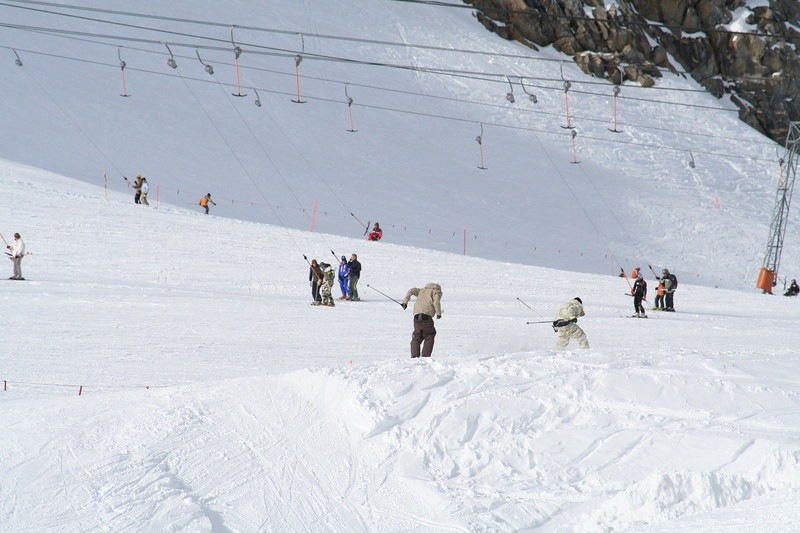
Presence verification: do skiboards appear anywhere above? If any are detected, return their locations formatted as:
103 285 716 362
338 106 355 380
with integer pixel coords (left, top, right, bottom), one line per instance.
626 315 649 318
0 278 26 280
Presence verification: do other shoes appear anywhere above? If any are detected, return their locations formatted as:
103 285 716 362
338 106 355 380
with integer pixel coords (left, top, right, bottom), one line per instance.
352 296 358 300
329 302 334 306
340 295 346 299
311 301 321 305
10 275 21 280
322 302 327 305
347 295 352 300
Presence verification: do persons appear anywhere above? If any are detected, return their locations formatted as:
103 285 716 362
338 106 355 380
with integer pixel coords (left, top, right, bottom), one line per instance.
661 269 678 312
367 222 382 242
132 175 150 205
553 296 590 349
760 282 777 295
199 193 216 215
631 267 641 278
654 281 666 310
632 273 647 318
402 282 443 359
784 278 800 297
6 233 26 280
309 254 361 307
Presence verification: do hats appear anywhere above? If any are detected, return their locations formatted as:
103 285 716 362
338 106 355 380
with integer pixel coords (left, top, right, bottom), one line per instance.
312 260 317 264
341 256 346 261
575 297 581 304
320 262 324 266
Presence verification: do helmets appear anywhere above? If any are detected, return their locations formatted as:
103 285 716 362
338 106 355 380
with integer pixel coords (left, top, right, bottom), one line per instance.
662 269 668 274
638 273 641 276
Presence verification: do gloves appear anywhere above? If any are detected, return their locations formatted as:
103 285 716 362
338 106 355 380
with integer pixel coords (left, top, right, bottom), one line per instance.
437 316 441 319
401 304 407 310
